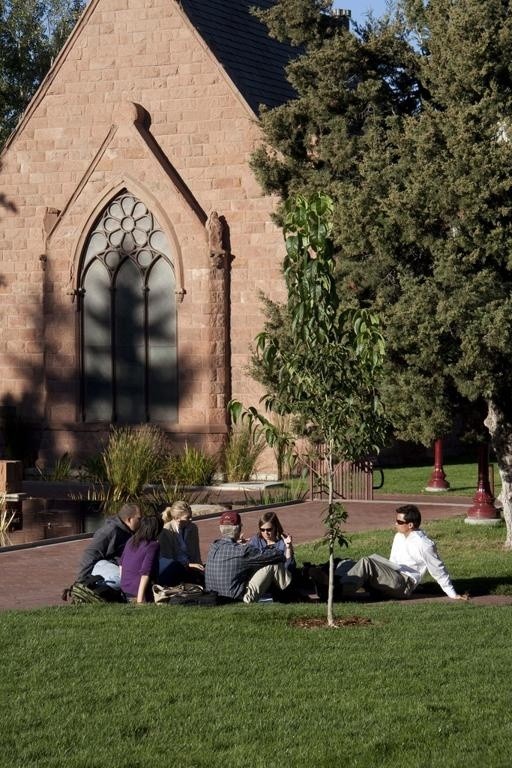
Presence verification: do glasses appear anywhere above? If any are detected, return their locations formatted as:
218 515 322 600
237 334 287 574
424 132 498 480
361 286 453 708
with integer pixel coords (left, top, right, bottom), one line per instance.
396 520 407 525
259 528 273 532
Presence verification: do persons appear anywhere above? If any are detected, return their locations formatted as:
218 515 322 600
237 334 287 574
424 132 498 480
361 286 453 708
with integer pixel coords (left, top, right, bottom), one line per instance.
154 500 204 587
237 511 295 600
203 510 312 604
74 502 142 592
308 503 469 601
119 514 164 605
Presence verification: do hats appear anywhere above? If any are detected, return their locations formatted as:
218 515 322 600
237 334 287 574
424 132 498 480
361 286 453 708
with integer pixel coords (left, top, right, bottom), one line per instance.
219 512 241 526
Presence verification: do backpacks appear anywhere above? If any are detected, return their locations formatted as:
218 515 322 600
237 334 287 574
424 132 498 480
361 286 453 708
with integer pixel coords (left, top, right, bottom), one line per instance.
63 575 128 603
152 584 217 604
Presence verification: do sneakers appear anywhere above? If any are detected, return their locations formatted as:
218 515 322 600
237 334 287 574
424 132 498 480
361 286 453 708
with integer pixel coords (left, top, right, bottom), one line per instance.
308 567 329 584
